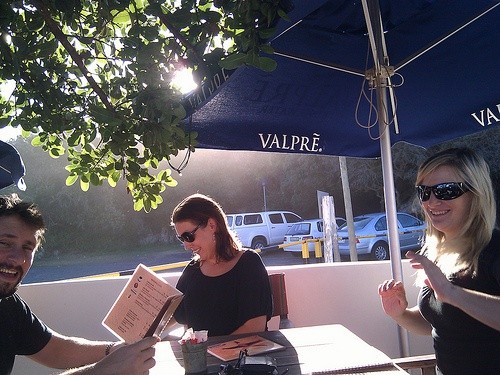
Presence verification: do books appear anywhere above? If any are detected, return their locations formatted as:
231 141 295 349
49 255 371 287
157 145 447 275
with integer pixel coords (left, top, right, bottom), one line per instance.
101 263 186 351
207 335 286 362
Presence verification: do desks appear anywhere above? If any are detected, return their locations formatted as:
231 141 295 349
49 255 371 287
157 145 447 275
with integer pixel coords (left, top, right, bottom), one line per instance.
149 323 410 375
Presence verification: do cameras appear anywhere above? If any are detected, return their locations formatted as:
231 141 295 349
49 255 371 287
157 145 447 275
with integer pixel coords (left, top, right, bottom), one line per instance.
240 357 278 375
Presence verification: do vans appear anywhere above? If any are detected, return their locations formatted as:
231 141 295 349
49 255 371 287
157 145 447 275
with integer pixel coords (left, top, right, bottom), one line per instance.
226 211 303 256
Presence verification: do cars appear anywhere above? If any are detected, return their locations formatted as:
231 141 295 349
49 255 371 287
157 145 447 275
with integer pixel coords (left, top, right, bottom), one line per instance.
337 212 428 260
283 218 346 255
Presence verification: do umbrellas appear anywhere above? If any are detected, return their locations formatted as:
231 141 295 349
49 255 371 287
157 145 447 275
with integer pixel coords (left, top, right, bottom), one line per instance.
144 0 500 356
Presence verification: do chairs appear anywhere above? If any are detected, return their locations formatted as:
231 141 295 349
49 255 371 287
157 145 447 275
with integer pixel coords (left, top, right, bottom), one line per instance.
268 274 289 330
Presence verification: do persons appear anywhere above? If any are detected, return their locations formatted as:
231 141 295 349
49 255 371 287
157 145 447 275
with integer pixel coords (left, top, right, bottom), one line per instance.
0 192 161 375
157 193 274 342
378 147 500 375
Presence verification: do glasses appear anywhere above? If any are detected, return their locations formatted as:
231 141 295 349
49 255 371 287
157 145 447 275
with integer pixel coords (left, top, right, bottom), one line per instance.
415 181 473 202
176 222 206 243
